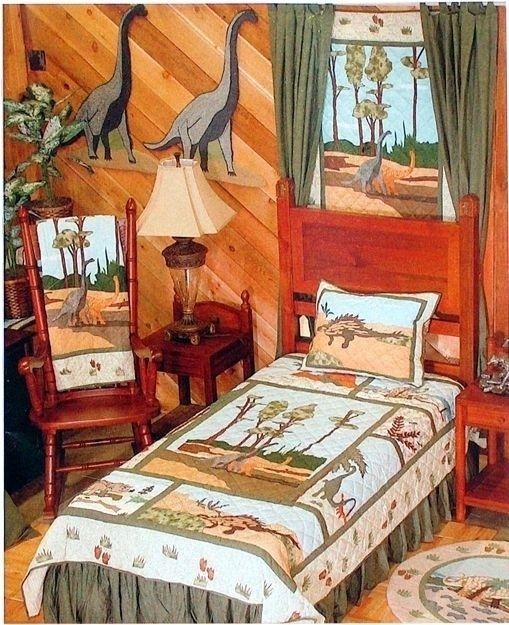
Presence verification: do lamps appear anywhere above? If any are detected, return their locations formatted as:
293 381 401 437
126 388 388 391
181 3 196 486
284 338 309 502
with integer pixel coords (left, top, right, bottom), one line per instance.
136 151 237 346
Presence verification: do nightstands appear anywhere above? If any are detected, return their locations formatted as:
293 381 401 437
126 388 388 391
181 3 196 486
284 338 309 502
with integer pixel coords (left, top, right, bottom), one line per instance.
141 286 255 444
454 379 509 524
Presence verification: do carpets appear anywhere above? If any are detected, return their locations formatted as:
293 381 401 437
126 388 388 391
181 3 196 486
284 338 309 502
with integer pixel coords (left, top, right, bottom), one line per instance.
386 537 509 623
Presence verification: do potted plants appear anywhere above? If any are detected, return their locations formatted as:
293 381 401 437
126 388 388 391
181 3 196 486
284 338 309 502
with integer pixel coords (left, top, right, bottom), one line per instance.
4 160 47 320
3 82 89 225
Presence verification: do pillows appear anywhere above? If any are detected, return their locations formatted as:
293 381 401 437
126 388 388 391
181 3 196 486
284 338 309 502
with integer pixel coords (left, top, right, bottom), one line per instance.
300 278 444 390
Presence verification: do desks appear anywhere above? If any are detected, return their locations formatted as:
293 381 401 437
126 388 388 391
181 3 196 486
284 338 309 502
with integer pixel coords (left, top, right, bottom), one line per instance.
4 316 45 506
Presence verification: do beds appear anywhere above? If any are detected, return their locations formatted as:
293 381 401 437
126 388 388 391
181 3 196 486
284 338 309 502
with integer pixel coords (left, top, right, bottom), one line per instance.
22 177 480 623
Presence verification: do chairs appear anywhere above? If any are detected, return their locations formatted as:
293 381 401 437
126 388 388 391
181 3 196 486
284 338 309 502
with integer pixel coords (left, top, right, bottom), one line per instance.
17 199 162 522
